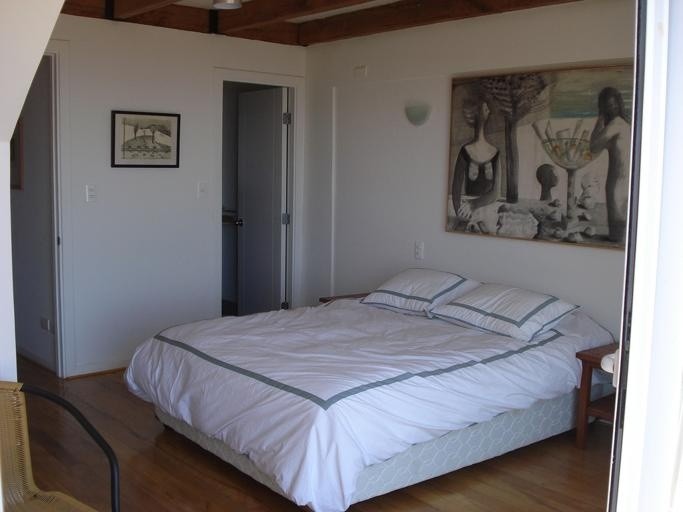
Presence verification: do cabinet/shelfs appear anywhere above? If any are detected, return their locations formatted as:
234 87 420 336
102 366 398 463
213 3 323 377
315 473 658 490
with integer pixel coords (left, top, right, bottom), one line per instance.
10 119 24 190
111 110 181 169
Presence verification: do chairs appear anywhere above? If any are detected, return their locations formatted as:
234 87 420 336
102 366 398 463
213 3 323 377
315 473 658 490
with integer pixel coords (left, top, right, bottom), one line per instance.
0 380 119 512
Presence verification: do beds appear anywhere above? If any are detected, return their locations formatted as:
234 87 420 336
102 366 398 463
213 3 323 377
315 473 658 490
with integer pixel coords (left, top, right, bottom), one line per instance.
122 276 615 512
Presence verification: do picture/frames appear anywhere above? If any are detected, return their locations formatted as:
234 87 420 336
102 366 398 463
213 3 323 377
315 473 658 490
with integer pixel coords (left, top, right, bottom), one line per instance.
444 53 633 250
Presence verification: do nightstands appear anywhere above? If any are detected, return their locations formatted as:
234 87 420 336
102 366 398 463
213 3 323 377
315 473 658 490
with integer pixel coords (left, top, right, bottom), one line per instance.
576 342 620 451
319 293 370 303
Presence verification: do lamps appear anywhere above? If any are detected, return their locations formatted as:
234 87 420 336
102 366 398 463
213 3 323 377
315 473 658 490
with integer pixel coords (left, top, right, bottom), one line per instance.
212 0 242 9
405 104 432 125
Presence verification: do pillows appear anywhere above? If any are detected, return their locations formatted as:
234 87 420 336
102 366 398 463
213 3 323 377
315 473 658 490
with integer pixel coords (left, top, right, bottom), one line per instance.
360 268 584 343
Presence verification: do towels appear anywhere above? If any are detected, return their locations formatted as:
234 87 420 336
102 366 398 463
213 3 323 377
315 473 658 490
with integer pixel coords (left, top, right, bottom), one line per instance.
601 348 620 387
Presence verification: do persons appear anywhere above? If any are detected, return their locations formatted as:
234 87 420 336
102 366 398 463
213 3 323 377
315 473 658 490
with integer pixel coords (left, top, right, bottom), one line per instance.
535 163 560 201
589 85 631 248
